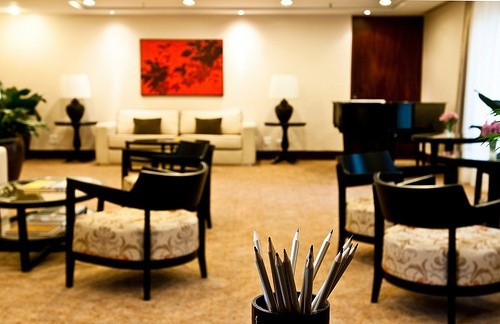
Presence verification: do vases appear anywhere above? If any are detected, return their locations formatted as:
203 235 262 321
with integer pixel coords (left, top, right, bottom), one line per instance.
488 144 498 159
442 127 454 137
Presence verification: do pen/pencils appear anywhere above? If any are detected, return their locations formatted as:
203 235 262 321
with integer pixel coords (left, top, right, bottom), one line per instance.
253 225 358 313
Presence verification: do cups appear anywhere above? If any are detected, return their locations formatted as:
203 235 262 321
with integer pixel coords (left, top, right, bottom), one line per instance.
251 292 330 324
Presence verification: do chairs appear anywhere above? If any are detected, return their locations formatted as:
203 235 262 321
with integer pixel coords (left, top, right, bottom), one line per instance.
370 171 500 324
121 140 209 191
335 157 449 259
65 160 209 300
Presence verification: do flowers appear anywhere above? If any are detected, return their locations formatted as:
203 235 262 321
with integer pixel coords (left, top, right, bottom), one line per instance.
468 93 500 159
481 119 500 150
440 112 458 131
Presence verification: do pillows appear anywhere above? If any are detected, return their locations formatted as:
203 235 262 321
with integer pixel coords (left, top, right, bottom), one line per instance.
132 118 161 133
195 118 222 135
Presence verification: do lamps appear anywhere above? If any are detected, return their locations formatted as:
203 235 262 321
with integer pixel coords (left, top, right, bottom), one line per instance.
60 74 92 122
268 75 299 123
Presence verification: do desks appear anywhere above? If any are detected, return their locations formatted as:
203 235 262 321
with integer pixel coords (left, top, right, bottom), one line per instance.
412 134 487 155
0 178 97 272
266 123 305 164
53 122 98 162
439 153 500 204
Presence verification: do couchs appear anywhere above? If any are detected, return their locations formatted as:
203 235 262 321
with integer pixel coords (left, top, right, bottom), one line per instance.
94 109 257 166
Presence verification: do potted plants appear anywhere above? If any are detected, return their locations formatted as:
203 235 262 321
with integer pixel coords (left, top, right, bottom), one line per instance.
0 80 49 180
